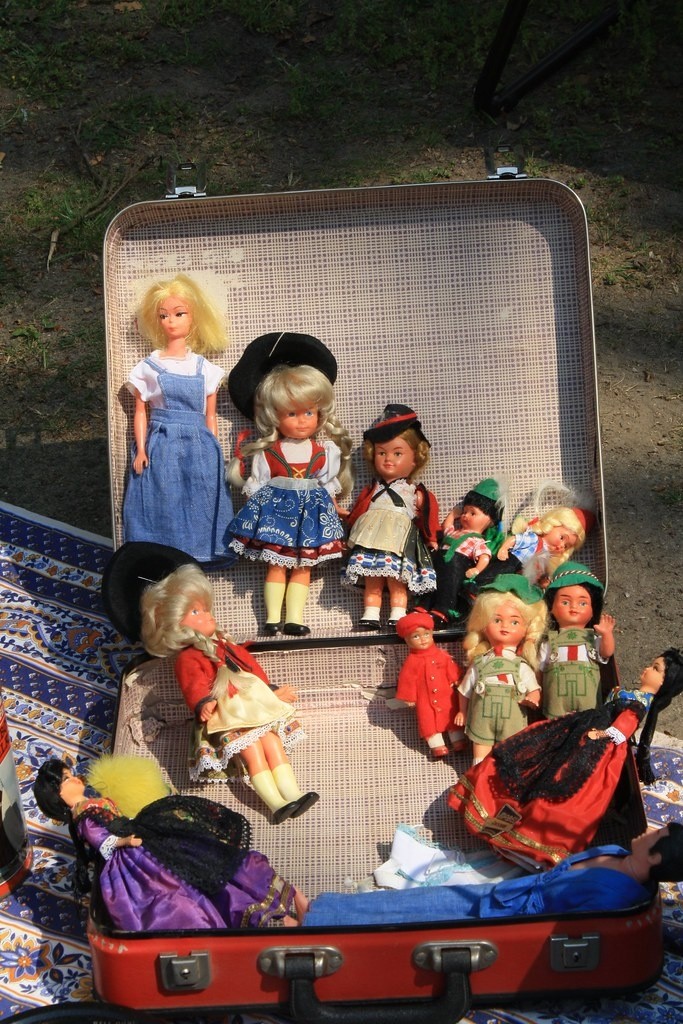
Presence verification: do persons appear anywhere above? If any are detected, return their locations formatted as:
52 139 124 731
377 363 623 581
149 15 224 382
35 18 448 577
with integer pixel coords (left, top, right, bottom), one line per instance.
453 574 548 765
122 275 238 571
413 479 596 630
536 561 617 721
340 403 441 628
224 332 356 636
284 823 683 926
446 647 683 874
396 613 468 757
34 758 297 932
102 541 320 825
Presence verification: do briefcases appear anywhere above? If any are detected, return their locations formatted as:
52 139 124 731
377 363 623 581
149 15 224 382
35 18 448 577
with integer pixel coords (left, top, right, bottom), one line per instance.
84 160 661 1023
